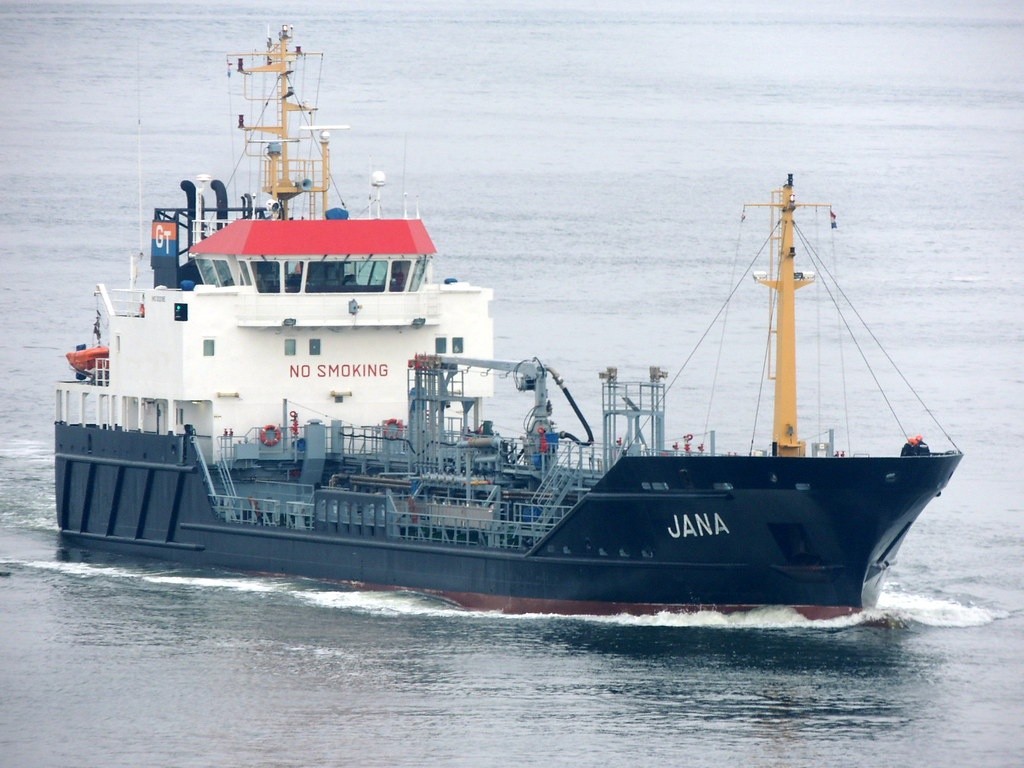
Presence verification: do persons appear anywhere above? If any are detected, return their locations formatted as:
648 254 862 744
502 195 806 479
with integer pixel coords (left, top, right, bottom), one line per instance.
900 433 931 456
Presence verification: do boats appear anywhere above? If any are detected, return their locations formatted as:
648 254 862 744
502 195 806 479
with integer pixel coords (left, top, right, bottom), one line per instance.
53 25 965 628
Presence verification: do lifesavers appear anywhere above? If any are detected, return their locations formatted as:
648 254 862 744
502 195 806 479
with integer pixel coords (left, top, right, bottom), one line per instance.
383 419 404 441
260 425 282 445
